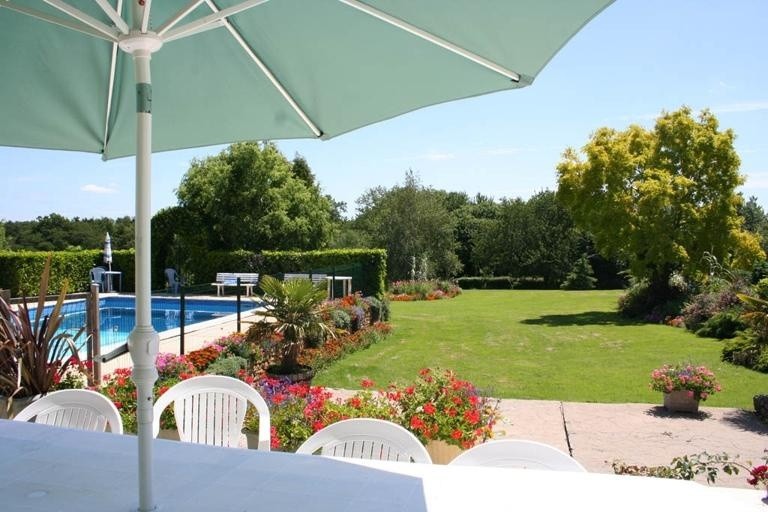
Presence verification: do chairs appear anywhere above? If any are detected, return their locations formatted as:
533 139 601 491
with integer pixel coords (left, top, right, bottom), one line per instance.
0 416 768 511
296 417 432 463
90 269 106 292
152 374 270 452
450 439 586 473
164 269 181 295
12 388 123 435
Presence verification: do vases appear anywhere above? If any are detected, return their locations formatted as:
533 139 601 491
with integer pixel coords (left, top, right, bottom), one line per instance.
664 390 700 414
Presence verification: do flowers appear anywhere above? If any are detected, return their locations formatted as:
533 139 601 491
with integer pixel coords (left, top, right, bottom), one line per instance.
649 360 720 399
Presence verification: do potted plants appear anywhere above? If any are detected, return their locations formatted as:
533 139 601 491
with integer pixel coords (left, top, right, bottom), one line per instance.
261 275 330 389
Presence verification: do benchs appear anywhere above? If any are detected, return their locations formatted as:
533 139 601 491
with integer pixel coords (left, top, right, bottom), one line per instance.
281 274 327 287
210 272 260 297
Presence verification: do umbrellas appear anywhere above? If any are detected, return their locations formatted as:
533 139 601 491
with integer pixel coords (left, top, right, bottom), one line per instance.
0 0 614 508
103 231 113 272
